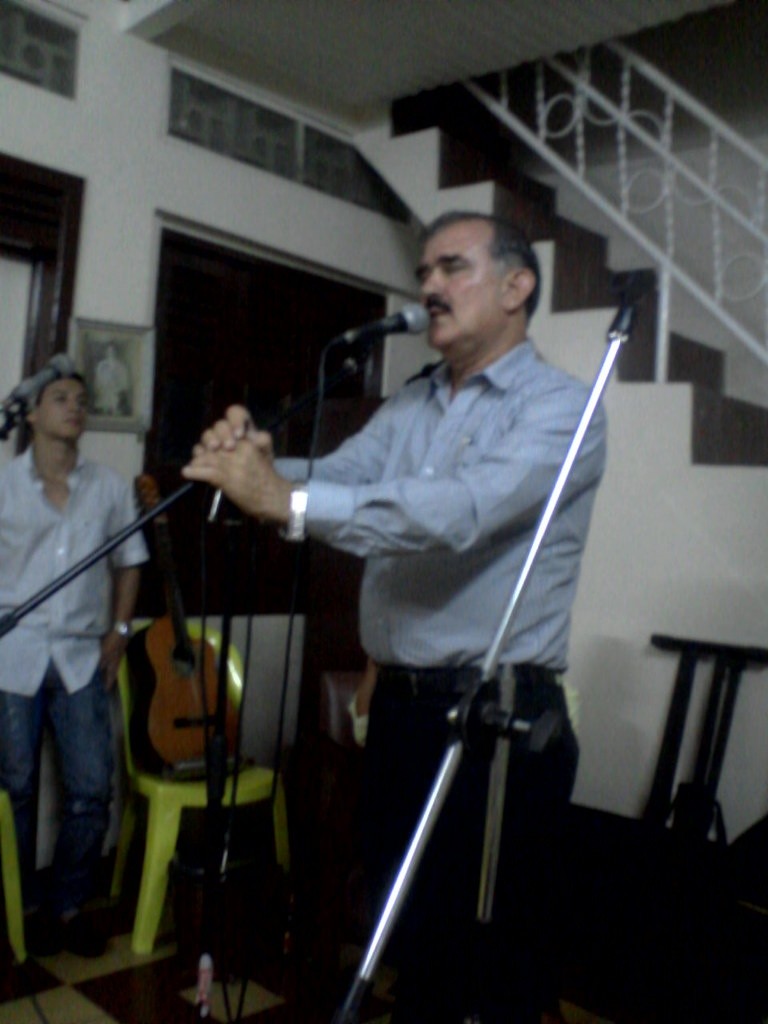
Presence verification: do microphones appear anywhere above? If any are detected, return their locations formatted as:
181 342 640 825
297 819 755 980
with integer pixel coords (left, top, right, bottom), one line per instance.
0 352 75 413
333 302 432 346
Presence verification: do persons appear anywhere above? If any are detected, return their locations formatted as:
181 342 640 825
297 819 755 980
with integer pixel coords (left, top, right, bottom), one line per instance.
179 209 607 1024
0 370 148 959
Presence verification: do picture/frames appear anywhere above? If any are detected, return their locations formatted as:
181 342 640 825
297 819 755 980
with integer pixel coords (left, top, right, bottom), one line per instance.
69 318 156 434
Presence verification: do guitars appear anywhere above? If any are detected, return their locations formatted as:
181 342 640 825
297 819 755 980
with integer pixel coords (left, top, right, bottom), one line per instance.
124 471 241 781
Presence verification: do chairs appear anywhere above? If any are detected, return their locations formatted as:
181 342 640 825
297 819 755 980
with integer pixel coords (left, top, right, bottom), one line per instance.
0 790 28 966
104 619 293 954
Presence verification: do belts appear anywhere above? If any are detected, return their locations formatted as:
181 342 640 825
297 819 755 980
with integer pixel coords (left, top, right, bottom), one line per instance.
381 665 557 696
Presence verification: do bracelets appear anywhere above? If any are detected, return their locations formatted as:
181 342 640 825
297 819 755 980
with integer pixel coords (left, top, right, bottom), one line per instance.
280 485 308 543
113 622 130 636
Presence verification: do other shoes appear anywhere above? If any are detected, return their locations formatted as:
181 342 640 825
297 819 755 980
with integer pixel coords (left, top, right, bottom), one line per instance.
20 905 47 956
53 908 113 956
